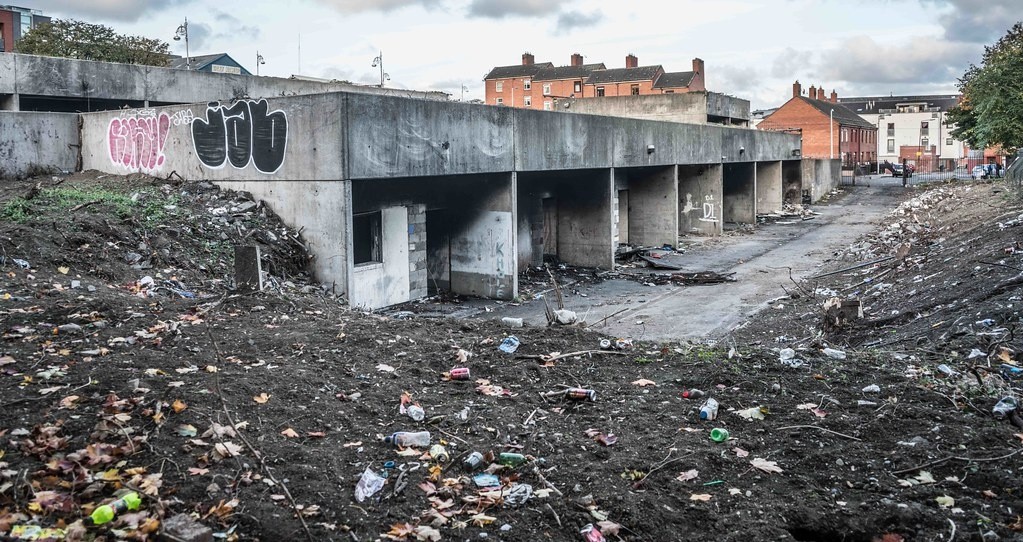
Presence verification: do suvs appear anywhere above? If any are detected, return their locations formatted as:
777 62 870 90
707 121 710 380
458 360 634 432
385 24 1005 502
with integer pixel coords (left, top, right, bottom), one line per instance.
971 164 1006 179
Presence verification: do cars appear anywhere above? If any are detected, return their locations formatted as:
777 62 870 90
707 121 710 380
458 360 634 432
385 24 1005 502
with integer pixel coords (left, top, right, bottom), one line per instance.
892 164 912 178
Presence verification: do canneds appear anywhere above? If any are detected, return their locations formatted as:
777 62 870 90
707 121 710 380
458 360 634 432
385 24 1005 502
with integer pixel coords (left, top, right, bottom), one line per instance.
992 396 1018 418
430 443 449 463
449 368 470 379
580 523 606 542
565 388 596 402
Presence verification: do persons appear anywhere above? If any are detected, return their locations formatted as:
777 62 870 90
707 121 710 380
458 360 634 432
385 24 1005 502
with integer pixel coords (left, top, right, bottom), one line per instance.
988 162 993 179
996 162 1001 178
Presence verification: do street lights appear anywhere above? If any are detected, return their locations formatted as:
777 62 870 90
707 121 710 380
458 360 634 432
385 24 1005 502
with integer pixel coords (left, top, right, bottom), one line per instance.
173 17 190 70
462 83 468 101
371 51 390 85
257 50 265 76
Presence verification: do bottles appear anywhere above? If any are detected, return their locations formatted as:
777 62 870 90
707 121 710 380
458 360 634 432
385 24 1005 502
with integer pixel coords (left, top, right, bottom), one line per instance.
0 242 1023 542
